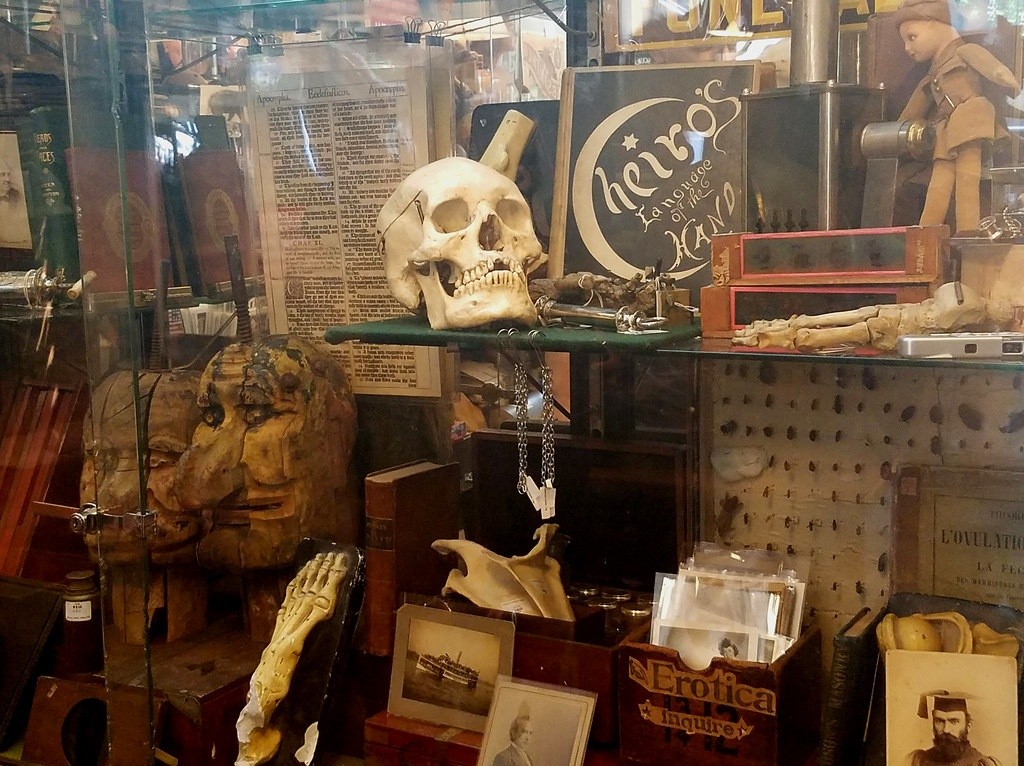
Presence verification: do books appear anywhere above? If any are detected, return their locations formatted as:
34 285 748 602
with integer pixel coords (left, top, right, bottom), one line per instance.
29 106 256 298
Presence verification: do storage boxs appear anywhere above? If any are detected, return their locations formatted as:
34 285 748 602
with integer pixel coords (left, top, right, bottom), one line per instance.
619 614 823 766
424 426 688 750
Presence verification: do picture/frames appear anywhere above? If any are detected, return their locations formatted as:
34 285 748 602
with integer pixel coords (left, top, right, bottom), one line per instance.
388 602 516 733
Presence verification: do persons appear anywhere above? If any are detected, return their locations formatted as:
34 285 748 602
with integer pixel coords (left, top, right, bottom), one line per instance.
80 370 207 646
718 638 739 661
911 690 1003 766
0 166 20 203
493 715 536 766
173 335 356 640
896 0 1021 234
